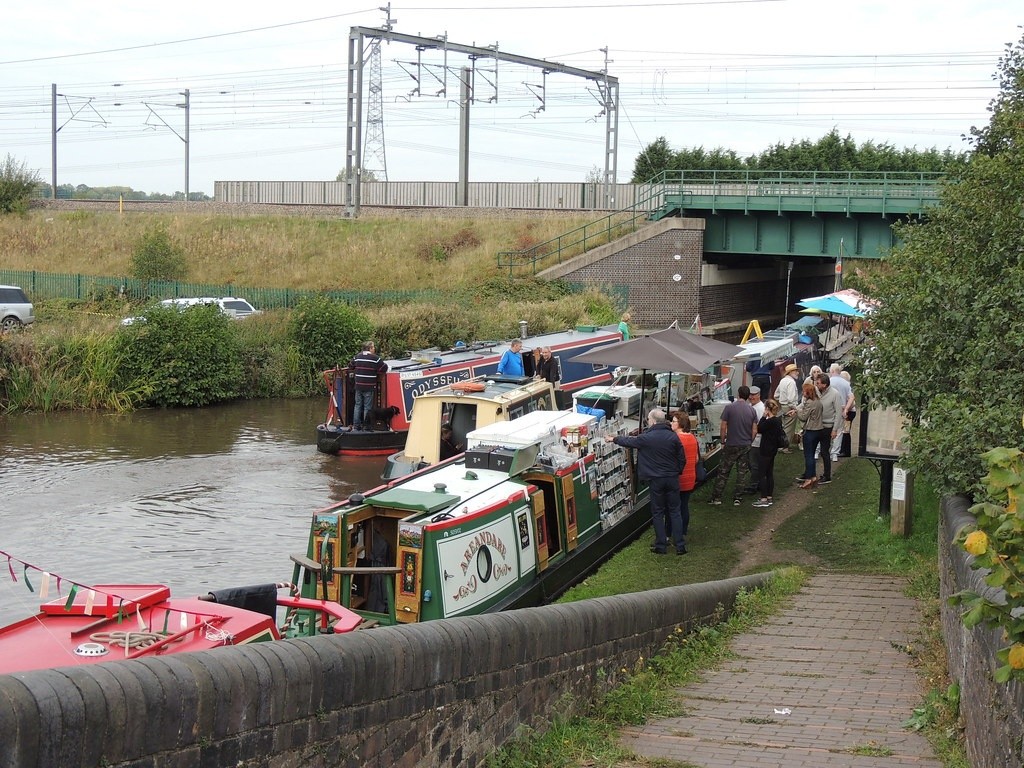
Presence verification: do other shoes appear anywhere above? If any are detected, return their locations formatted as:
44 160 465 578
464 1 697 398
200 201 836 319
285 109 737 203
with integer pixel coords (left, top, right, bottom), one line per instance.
354 424 361 430
829 453 839 461
678 545 688 554
650 544 666 553
798 443 804 451
837 451 849 457
814 453 818 460
777 447 793 454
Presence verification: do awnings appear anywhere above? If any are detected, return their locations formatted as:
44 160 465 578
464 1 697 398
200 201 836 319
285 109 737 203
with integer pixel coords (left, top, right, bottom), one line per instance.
731 335 797 368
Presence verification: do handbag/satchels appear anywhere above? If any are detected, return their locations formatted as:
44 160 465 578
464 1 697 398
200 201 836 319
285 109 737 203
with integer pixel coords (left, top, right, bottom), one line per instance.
841 418 851 434
776 416 789 448
696 441 704 482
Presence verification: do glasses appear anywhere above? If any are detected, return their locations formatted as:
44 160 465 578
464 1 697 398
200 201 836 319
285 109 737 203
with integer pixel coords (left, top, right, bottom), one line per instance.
672 420 678 423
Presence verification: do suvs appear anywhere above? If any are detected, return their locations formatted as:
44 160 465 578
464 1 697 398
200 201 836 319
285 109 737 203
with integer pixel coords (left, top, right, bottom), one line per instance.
121 296 254 327
0 285 36 334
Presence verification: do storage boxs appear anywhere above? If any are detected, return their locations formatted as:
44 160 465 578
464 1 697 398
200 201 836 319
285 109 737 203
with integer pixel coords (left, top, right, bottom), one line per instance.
575 395 620 419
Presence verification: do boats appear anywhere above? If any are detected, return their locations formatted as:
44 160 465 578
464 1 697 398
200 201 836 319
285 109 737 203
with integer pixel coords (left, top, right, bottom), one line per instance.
318 319 624 455
283 286 878 633
0 568 362 677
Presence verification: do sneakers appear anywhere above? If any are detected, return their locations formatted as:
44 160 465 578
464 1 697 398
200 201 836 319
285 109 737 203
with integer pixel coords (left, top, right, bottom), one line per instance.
817 473 832 484
706 498 721 505
752 497 769 507
733 499 740 506
757 497 773 505
796 473 813 480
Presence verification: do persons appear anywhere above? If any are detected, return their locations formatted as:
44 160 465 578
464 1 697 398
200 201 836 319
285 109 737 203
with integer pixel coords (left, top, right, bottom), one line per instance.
495 337 561 392
604 409 687 554
660 413 699 544
618 314 630 341
440 425 463 461
349 340 388 430
707 364 856 507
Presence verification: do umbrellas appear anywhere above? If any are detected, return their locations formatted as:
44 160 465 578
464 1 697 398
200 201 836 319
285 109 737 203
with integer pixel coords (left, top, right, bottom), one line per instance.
796 287 884 367
568 326 747 433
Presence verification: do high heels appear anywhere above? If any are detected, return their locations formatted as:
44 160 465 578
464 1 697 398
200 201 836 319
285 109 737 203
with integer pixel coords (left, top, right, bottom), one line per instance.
798 475 818 489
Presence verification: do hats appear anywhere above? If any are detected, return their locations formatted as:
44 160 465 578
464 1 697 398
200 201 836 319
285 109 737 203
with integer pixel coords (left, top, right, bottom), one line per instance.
784 364 800 376
748 386 760 394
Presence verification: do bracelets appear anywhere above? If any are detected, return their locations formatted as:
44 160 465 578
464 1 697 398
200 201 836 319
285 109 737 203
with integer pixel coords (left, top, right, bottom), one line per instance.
497 371 502 373
